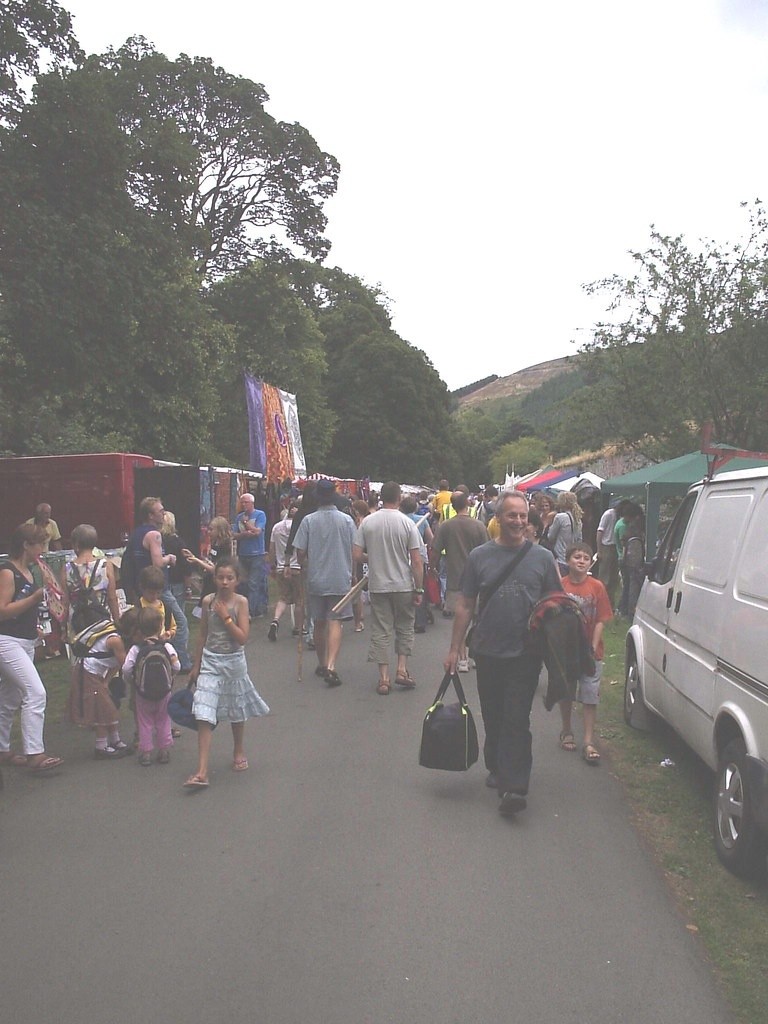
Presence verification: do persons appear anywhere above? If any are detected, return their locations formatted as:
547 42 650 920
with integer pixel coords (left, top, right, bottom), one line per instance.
134 566 182 738
121 606 182 767
0 524 65 772
442 491 568 814
21 479 659 694
166 555 271 788
58 522 123 729
560 540 615 763
70 606 142 762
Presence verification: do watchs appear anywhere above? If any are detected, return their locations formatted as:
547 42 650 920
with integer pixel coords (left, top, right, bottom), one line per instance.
242 520 248 524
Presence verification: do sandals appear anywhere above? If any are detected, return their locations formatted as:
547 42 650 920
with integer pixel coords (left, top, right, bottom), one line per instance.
559 730 577 751
315 665 328 676
324 670 342 685
377 679 392 696
395 670 416 687
583 746 600 760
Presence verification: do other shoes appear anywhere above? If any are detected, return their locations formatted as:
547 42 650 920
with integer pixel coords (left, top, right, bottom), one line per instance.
291 627 299 637
95 739 135 758
486 771 498 787
354 622 364 631
498 791 528 814
457 658 471 672
171 728 180 738
267 620 279 642
138 754 153 765
157 750 169 763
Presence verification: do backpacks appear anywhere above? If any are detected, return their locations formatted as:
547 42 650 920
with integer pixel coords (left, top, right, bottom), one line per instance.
132 639 174 701
70 620 123 659
622 528 645 568
69 559 110 630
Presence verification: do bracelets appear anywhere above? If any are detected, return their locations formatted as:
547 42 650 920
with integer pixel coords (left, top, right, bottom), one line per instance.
223 616 233 625
284 564 290 567
415 588 424 593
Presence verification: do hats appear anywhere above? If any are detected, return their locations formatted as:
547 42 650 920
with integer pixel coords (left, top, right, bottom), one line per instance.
311 480 340 500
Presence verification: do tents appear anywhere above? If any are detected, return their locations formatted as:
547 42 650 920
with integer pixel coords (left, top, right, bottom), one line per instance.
496 441 768 561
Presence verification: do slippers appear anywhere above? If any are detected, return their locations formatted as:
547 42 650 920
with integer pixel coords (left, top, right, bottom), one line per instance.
231 755 249 770
0 752 29 769
181 774 209 786
30 756 65 772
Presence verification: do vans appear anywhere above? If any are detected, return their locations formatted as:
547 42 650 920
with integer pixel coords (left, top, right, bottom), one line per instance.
624 462 767 870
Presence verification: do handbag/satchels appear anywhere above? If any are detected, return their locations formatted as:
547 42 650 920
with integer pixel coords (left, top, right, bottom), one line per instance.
108 676 128 698
422 569 441 606
167 674 219 732
418 670 479 772
